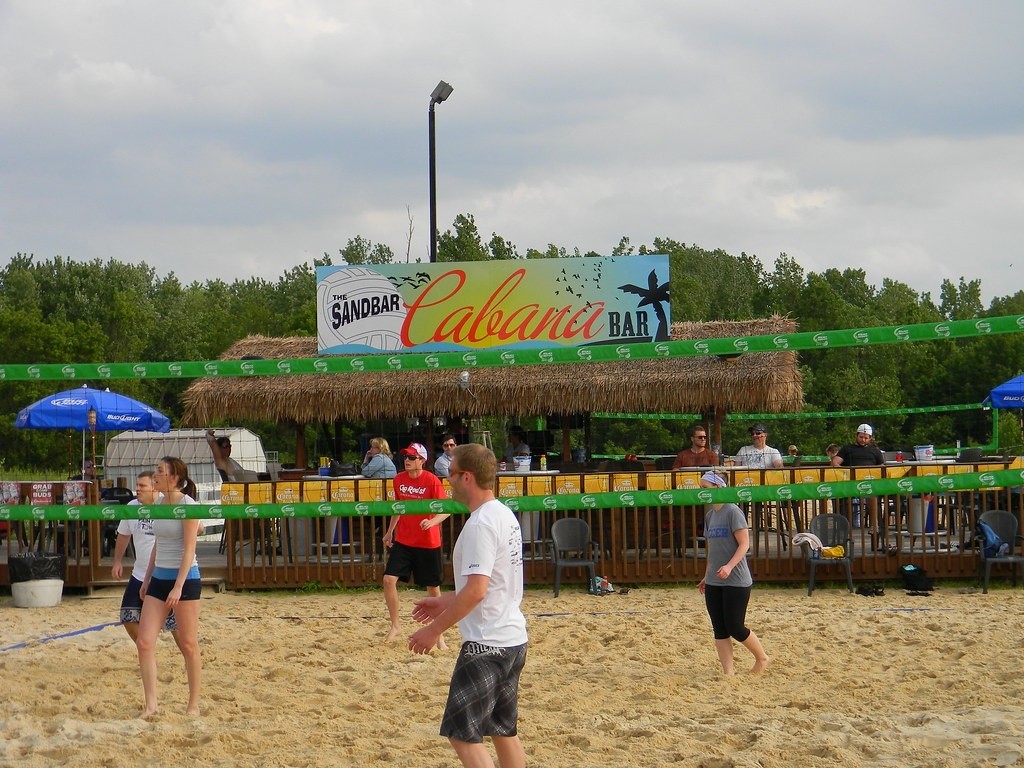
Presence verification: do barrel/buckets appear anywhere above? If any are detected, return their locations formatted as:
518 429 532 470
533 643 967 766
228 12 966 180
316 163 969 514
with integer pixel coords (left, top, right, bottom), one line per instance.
914 445 933 461
513 457 531 471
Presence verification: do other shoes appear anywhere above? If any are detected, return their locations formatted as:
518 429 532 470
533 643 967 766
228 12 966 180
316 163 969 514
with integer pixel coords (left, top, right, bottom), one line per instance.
868 525 880 534
956 587 979 594
906 591 931 597
619 587 629 595
940 541 958 553
882 543 897 555
855 582 884 597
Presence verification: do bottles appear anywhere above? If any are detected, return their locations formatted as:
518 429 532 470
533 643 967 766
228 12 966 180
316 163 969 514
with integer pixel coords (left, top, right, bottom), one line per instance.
540 455 546 470
601 576 608 594
500 462 506 472
896 451 902 462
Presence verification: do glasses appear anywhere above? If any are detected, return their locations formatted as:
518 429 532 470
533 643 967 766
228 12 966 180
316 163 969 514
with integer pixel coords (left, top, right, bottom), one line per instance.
695 435 707 440
447 467 474 477
370 446 378 450
750 431 763 435
87 463 94 468
222 444 231 448
443 443 456 448
402 455 422 461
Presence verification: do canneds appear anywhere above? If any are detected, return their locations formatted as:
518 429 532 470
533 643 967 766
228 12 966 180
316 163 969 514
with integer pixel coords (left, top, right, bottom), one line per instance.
896 450 903 461
813 549 819 560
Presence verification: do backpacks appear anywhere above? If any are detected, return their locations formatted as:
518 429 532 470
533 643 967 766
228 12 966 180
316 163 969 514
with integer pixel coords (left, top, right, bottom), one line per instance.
898 562 935 591
975 517 1009 559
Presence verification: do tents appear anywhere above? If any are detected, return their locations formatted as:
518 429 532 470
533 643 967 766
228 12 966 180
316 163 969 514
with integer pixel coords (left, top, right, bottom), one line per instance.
983 373 1024 455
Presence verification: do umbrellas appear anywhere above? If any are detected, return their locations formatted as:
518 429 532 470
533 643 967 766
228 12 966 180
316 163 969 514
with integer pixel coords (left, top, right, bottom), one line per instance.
16 383 170 479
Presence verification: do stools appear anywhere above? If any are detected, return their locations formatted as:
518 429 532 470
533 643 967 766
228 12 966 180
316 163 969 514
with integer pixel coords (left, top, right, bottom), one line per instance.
217 468 293 565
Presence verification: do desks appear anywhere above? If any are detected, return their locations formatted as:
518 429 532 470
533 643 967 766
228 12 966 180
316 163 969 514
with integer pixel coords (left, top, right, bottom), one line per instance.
0 487 137 559
678 465 752 559
55 499 120 560
301 473 367 560
496 468 560 555
885 459 957 554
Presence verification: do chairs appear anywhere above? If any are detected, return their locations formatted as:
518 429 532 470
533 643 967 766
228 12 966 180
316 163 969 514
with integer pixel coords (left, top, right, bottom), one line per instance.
552 518 599 598
806 514 854 596
976 509 1024 593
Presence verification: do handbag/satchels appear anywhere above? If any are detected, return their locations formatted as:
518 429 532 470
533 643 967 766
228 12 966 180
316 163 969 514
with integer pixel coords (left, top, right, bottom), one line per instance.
329 459 357 477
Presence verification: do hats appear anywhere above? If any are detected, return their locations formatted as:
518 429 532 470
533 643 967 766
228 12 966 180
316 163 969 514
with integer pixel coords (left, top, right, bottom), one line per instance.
400 442 427 461
788 445 797 452
748 422 768 433
856 424 872 436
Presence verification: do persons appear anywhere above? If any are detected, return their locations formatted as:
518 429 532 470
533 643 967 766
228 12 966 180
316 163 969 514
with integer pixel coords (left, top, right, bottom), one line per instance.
674 425 720 470
789 445 799 456
500 426 529 462
205 429 244 482
112 456 205 717
826 424 885 534
70 457 102 491
734 422 783 470
361 437 397 538
408 444 530 768
383 442 452 649
696 470 771 676
434 433 458 478
